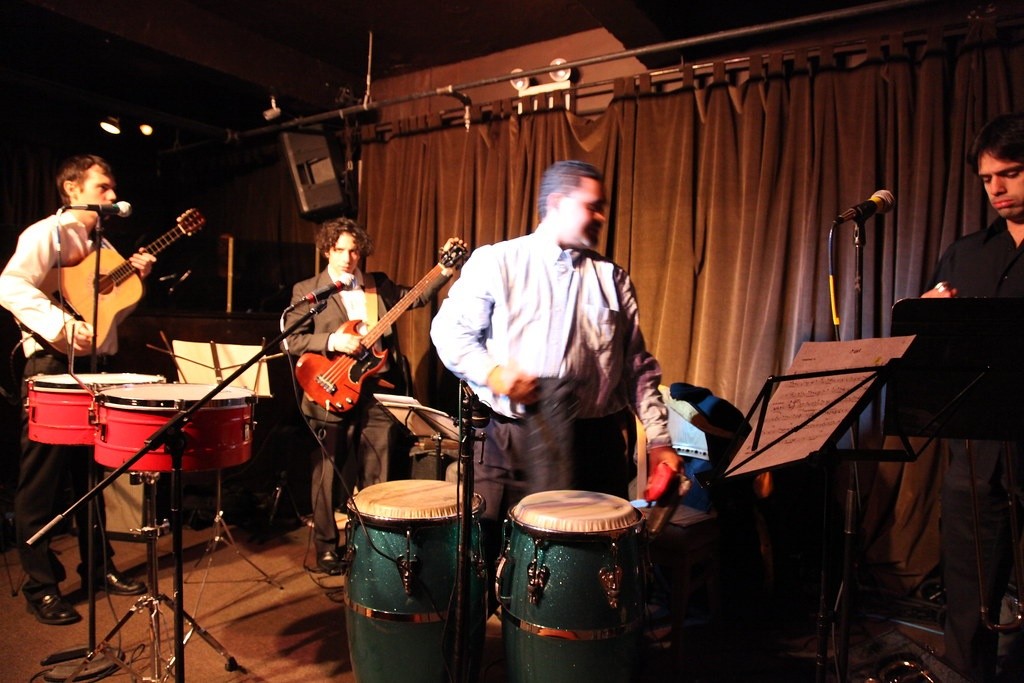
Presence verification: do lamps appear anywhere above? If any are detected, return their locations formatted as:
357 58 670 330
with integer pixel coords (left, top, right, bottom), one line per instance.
263 95 282 122
99 111 120 134
510 59 574 111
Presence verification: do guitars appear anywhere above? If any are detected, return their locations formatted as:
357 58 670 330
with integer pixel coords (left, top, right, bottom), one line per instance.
294 241 468 415
13 207 205 362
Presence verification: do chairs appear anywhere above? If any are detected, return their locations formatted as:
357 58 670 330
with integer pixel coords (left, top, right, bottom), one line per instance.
644 414 744 683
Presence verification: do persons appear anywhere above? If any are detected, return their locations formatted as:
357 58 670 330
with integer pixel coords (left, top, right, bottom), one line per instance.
917 108 1024 683
430 159 682 624
0 152 156 626
289 215 469 575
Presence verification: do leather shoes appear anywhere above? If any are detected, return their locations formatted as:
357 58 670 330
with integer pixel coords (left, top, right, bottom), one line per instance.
318 549 343 575
82 564 148 596
26 594 80 625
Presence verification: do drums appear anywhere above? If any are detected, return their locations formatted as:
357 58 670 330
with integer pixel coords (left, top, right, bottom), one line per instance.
341 478 491 683
497 489 646 683
89 380 259 472
26 371 166 446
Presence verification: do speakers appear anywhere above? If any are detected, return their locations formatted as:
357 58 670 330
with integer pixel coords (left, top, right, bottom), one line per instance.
280 123 343 214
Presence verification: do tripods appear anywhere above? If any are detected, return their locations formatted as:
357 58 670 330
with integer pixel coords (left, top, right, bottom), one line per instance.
183 470 284 626
63 471 258 683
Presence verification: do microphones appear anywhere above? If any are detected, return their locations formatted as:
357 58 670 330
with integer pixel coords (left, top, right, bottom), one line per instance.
461 378 490 428
284 272 357 314
831 189 895 226
64 201 132 218
